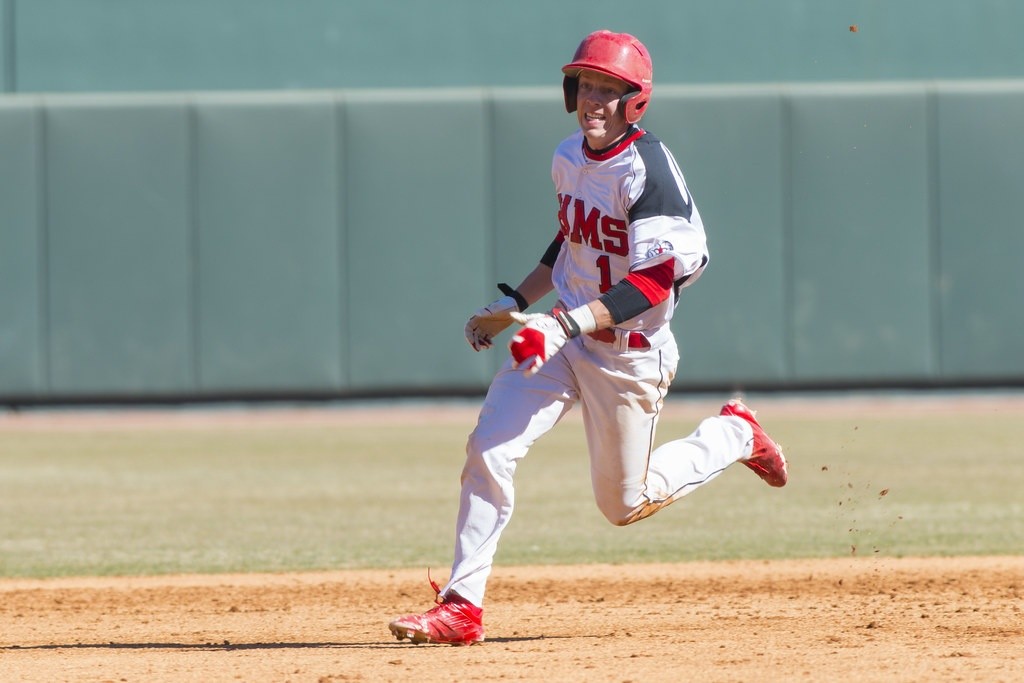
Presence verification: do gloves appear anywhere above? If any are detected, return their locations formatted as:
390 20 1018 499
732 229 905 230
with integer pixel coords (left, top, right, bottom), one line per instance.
465 295 520 353
508 304 581 379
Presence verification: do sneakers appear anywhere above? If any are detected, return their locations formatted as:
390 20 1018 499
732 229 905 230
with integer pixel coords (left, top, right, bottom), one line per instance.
388 566 485 646
720 397 787 487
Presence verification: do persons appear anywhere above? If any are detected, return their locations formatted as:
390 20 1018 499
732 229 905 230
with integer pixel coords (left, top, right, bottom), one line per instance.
388 30 787 645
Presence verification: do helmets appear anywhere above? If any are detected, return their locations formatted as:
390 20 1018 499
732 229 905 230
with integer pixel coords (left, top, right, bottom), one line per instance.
561 30 653 123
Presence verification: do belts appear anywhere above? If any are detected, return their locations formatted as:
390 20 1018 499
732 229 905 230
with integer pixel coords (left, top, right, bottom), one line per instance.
551 300 651 348
